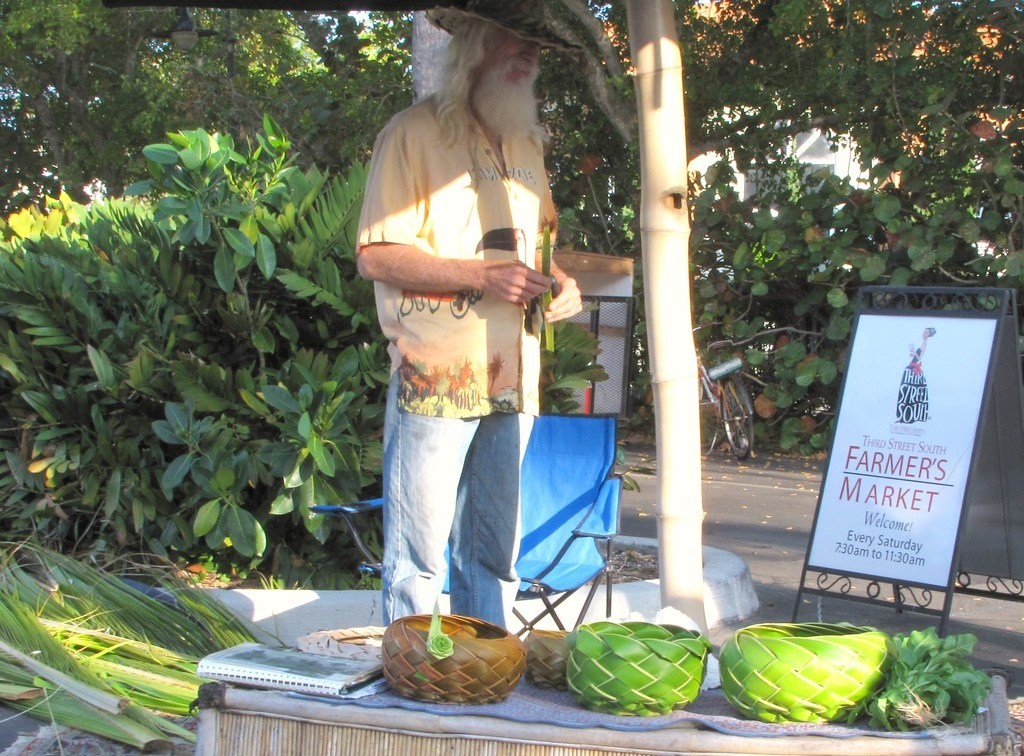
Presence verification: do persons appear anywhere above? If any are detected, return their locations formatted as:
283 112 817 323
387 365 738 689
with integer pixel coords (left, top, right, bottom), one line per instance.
355 0 582 627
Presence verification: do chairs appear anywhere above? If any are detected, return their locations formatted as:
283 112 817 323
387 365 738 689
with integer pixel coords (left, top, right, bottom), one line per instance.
310 411 622 642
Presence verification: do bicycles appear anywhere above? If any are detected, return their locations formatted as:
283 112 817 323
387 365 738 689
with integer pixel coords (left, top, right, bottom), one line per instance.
693 323 756 460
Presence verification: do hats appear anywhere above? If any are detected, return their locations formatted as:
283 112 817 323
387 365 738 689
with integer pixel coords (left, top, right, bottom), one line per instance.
425 0 584 53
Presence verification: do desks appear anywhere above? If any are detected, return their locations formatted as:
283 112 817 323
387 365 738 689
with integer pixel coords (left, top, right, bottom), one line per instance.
187 668 1022 756
